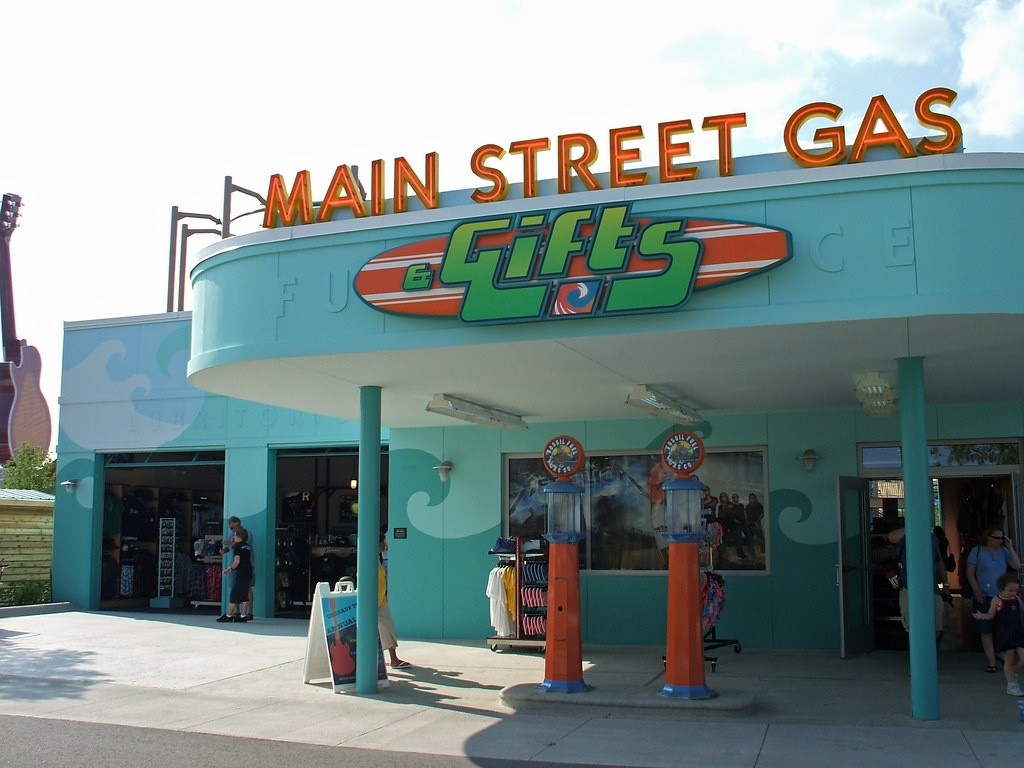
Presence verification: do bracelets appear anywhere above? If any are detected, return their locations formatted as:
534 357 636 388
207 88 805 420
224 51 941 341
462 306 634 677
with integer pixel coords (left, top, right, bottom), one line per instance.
231 567 234 571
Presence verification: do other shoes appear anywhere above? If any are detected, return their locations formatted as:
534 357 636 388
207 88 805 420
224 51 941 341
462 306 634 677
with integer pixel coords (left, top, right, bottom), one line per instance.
232 613 241 617
243 614 253 620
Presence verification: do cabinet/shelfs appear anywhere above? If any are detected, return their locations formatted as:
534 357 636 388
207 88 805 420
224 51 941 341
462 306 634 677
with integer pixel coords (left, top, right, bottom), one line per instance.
485 548 546 652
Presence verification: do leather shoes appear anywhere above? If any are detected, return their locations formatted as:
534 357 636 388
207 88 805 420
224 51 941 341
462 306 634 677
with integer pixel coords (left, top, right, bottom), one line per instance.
216 613 234 622
234 615 247 623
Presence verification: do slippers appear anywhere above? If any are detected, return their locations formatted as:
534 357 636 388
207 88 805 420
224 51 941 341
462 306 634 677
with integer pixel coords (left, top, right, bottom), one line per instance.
986 666 997 672
391 661 412 669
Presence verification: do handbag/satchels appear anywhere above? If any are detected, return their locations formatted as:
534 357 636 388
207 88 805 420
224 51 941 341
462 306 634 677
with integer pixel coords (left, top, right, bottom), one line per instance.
946 545 956 572
962 543 980 600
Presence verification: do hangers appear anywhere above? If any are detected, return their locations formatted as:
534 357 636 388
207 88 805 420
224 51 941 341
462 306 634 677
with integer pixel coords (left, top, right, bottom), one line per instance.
495 562 515 568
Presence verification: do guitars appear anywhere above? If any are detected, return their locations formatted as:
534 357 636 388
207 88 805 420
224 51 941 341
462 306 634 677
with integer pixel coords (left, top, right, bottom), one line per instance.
0 192 52 469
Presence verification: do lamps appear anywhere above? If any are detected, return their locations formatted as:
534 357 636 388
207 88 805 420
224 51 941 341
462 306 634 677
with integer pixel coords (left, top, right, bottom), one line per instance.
425 393 527 430
434 461 453 481
625 384 702 424
795 450 818 473
858 373 894 418
59 480 75 486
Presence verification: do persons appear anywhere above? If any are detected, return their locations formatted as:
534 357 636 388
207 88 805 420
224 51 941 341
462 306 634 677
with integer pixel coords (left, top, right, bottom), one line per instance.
972 574 1024 698
691 475 764 561
216 528 253 623
964 526 1022 673
378 527 412 668
216 516 255 623
889 525 956 675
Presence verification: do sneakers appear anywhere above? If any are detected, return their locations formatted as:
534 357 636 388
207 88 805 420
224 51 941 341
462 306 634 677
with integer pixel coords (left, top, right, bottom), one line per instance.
1006 687 1024 696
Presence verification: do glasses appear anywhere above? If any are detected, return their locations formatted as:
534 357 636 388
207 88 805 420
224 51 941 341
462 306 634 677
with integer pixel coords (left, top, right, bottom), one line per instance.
990 536 1005 540
230 527 234 530
